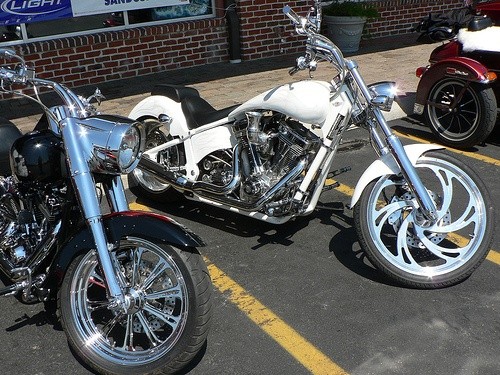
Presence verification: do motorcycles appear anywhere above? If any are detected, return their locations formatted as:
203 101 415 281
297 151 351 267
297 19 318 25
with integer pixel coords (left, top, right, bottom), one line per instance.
0 47 214 375
126 0 497 290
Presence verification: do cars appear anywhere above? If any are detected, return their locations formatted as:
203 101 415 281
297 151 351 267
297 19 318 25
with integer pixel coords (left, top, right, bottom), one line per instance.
103 12 135 28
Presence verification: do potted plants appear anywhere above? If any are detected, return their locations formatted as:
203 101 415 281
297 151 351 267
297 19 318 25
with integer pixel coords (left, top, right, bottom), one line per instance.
321 0 379 51
